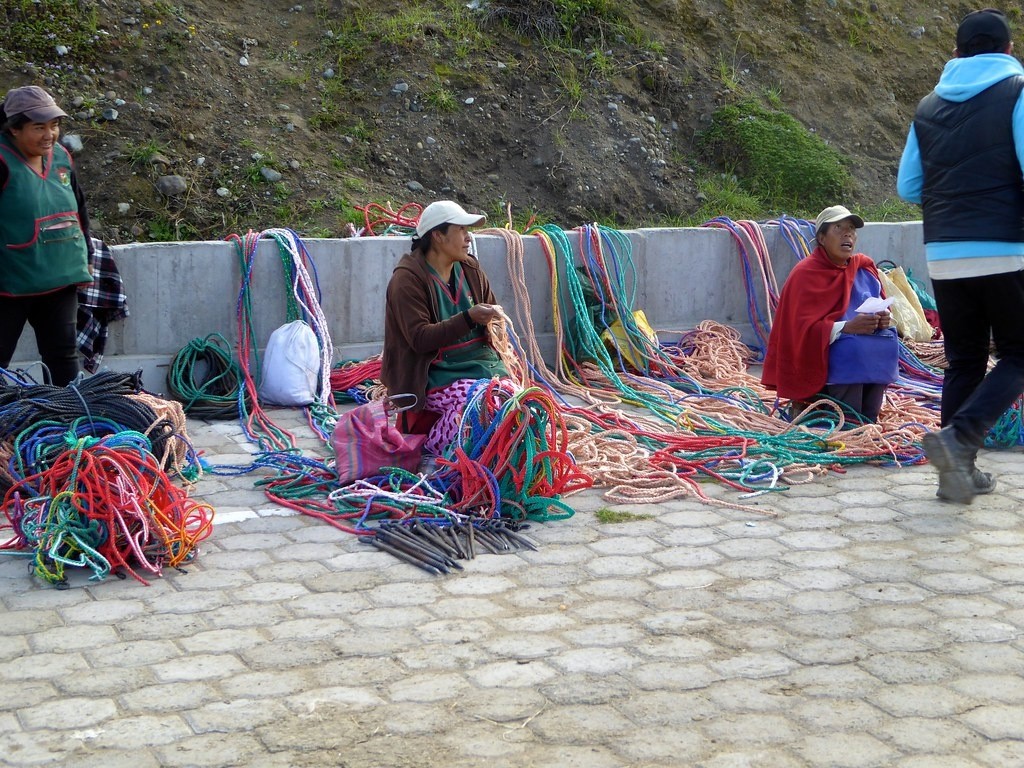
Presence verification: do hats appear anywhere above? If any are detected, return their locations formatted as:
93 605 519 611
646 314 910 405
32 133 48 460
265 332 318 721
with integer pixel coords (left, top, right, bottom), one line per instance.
416 200 486 239
3 86 68 123
957 8 1011 57
816 205 864 235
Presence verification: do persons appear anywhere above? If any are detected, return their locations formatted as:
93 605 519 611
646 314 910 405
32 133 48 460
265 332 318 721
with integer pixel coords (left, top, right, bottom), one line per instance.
760 205 899 424
379 201 512 480
0 85 95 387
896 7 1024 506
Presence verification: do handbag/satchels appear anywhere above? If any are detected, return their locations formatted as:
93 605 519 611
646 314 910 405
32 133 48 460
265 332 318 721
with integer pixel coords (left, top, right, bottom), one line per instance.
329 393 427 487
877 266 937 342
257 319 321 407
875 260 942 340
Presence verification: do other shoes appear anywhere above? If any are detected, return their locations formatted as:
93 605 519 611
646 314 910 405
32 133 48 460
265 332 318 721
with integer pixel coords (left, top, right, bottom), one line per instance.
923 424 979 505
936 461 997 498
